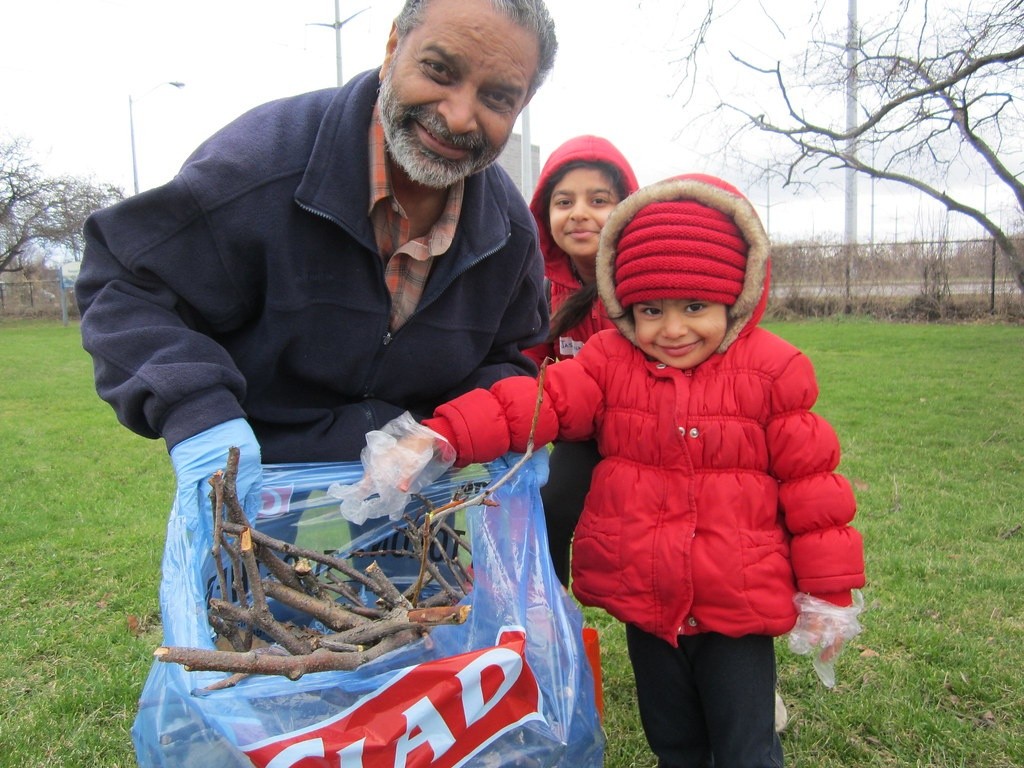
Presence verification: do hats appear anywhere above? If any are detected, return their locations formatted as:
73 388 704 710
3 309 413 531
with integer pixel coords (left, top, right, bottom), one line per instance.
614 201 748 309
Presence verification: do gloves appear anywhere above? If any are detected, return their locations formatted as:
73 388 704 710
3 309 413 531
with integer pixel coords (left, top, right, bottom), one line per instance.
483 447 550 495
170 418 261 550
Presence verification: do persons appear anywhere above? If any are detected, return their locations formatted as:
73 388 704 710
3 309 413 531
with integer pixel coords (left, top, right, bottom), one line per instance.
73 0 559 631
522 137 640 593
359 171 868 767
